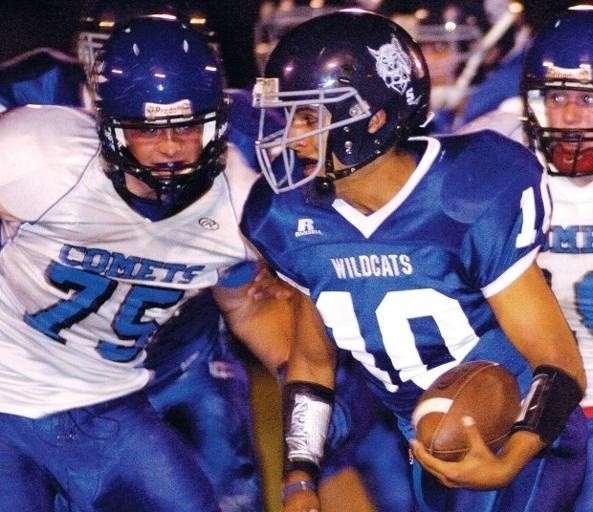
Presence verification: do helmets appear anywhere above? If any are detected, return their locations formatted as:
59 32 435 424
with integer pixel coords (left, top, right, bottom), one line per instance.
251 8 431 196
521 5 593 176
90 14 229 209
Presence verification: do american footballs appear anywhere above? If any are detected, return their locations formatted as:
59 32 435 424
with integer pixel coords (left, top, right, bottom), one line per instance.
410 361 520 462
